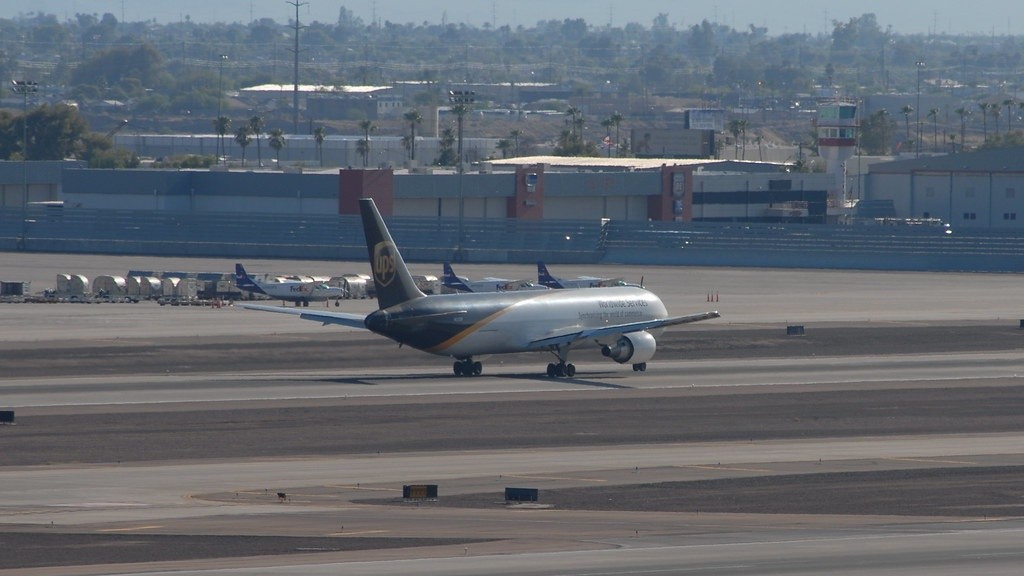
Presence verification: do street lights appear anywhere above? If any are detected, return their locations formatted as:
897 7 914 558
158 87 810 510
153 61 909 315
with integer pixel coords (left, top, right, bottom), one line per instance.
215 54 229 166
11 79 39 250
448 88 477 262
913 61 926 157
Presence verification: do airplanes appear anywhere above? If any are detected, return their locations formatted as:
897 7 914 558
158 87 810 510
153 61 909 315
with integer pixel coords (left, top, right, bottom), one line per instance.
233 197 721 380
